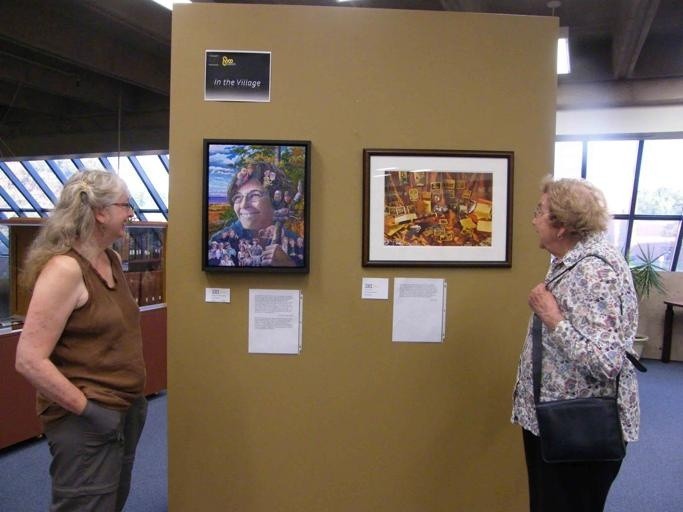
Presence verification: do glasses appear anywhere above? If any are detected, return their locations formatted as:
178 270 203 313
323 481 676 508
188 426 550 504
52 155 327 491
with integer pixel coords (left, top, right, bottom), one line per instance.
232 190 269 203
534 207 551 218
112 202 134 211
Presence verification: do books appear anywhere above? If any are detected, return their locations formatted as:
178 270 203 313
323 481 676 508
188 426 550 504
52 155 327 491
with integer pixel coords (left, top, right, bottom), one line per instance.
111 227 165 307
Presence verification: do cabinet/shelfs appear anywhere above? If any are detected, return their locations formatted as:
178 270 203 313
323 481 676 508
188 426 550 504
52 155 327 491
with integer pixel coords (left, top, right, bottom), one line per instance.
0 218 168 330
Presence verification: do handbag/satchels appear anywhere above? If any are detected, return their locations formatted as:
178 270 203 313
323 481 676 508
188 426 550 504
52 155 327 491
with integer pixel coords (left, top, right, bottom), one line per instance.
531 395 627 465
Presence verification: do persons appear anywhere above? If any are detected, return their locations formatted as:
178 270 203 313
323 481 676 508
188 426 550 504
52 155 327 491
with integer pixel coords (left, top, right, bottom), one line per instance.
13 167 149 512
207 160 306 266
509 176 640 510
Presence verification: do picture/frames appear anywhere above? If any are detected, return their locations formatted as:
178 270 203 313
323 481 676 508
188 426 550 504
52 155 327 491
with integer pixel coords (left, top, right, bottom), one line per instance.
201 138 311 275
362 148 513 269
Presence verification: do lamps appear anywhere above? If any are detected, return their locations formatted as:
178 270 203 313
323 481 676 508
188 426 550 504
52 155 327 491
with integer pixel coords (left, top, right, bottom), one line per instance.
547 1 570 75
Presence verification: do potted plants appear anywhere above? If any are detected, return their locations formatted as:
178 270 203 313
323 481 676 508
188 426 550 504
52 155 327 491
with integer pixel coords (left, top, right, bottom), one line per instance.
621 243 670 358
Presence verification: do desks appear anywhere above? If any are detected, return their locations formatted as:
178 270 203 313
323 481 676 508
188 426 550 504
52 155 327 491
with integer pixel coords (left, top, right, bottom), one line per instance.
662 297 683 363
0 302 167 450
